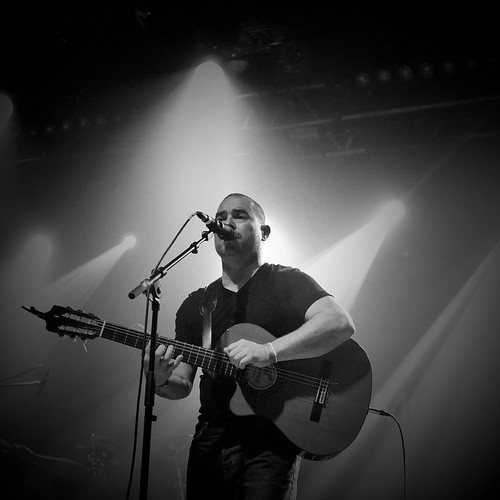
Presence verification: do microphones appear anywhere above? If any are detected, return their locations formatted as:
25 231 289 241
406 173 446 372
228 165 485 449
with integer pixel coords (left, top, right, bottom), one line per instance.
196 212 234 240
36 367 50 397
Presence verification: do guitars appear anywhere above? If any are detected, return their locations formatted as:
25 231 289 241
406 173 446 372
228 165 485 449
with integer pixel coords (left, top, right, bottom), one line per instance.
21 305 374 460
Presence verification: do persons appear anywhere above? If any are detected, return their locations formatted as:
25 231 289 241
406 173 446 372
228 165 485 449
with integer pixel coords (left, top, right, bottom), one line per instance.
144 193 355 500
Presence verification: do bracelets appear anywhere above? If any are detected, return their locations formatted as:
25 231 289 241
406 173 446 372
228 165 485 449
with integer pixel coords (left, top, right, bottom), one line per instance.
264 342 277 365
